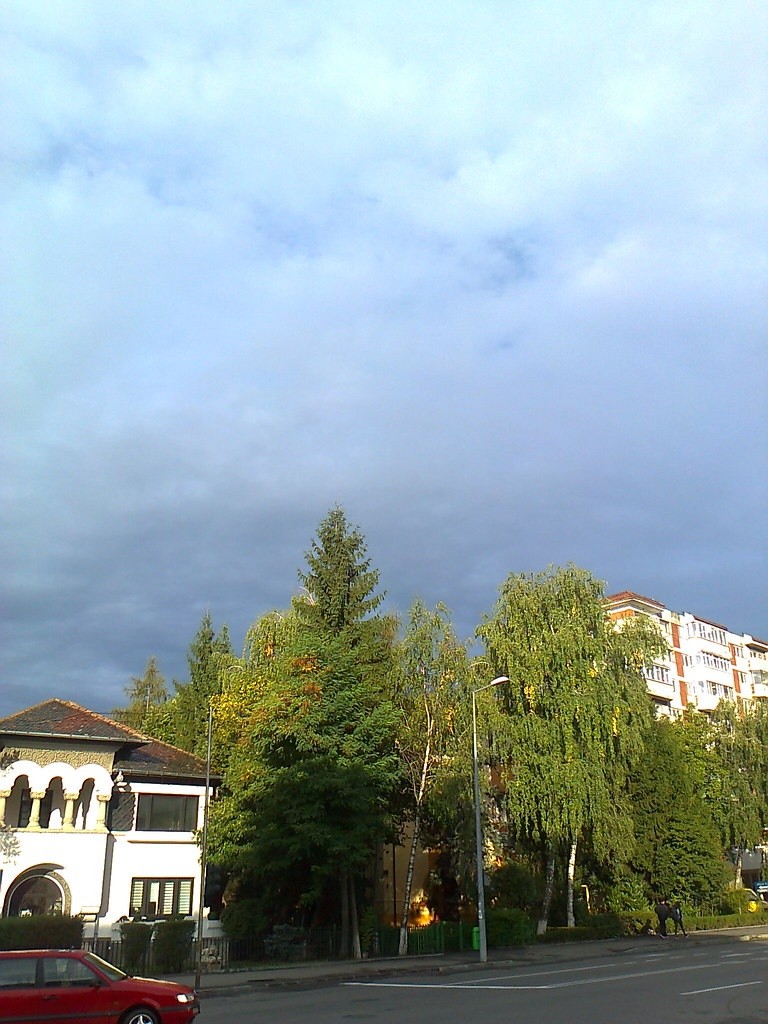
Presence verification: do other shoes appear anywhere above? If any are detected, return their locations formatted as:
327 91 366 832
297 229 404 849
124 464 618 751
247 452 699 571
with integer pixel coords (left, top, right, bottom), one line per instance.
675 935 678 939
685 934 689 939
660 934 668 938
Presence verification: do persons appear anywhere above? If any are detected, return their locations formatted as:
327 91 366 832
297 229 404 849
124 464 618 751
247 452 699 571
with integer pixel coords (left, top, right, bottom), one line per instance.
655 897 670 939
671 901 690 938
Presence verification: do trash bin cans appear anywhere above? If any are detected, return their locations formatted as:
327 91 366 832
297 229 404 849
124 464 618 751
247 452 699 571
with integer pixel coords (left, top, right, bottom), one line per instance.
471 926 480 951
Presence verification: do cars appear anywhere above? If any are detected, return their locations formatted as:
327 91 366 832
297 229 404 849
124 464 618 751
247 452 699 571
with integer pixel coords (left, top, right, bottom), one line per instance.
0 950 202 1024
